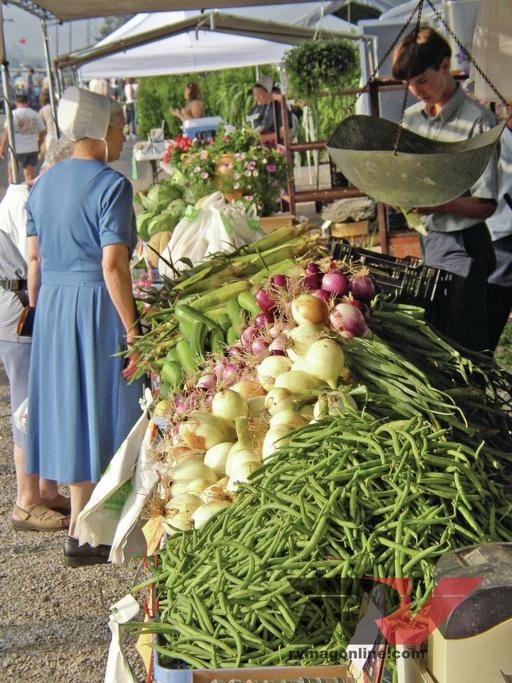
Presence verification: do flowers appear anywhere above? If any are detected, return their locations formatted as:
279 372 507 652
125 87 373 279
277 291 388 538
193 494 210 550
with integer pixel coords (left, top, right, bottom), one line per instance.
164 128 292 216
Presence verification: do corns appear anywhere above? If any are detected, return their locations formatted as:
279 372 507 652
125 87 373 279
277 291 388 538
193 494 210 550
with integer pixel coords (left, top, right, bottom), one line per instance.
108 218 322 385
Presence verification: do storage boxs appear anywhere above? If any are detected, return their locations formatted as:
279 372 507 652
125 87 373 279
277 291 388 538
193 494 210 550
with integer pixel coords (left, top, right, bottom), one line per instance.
179 116 223 140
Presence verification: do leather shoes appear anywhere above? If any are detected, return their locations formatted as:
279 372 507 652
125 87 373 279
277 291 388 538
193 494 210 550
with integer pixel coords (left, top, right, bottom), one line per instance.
63 536 111 567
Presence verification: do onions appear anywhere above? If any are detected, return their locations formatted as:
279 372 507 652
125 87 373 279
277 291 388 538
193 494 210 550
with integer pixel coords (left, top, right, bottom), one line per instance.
151 289 355 500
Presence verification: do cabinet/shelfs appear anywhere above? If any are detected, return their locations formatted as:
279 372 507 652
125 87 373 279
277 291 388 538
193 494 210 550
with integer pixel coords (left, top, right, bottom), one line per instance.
271 71 469 227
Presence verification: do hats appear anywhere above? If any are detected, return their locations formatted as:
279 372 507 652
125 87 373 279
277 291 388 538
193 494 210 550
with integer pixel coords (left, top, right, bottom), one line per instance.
57 85 110 141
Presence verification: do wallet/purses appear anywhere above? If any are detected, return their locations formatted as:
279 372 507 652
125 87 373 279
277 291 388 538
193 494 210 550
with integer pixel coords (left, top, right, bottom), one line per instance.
17 307 35 336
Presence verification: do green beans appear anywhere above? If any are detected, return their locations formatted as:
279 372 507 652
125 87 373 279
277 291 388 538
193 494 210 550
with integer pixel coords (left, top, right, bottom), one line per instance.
132 402 512 669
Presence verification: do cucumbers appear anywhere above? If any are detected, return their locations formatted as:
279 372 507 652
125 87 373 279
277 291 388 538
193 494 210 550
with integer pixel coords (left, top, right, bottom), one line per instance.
155 288 264 399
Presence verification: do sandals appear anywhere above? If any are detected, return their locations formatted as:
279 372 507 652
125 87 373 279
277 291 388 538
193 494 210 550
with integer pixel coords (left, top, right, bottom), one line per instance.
12 495 70 532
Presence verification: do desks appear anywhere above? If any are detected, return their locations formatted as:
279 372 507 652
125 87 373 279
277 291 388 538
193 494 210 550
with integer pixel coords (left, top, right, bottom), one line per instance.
160 160 176 176
133 139 176 183
260 214 296 234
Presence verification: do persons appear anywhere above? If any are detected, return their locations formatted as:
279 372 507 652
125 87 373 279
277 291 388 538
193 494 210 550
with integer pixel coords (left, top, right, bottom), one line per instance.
393 28 503 354
23 82 143 566
1 63 295 189
0 150 68 530
